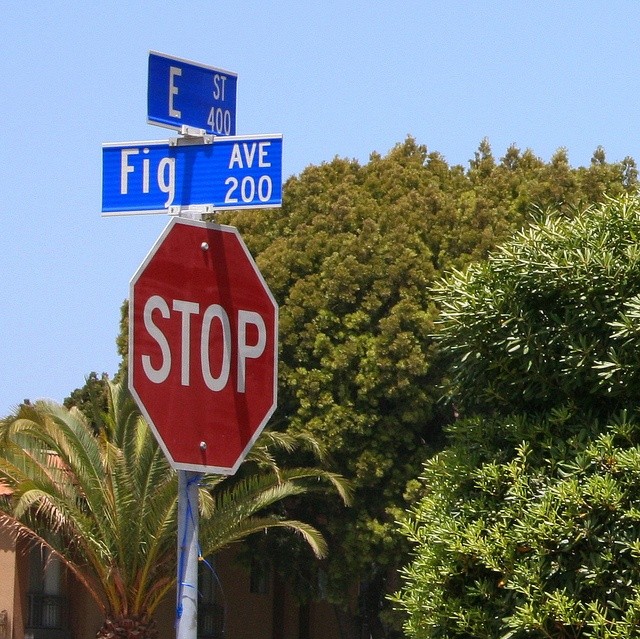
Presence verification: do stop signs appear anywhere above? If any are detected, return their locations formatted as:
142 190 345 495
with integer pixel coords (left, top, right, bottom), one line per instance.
127 216 278 475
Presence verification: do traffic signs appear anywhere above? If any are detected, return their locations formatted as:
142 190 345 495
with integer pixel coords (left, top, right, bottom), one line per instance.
100 133 282 216
146 50 237 137
